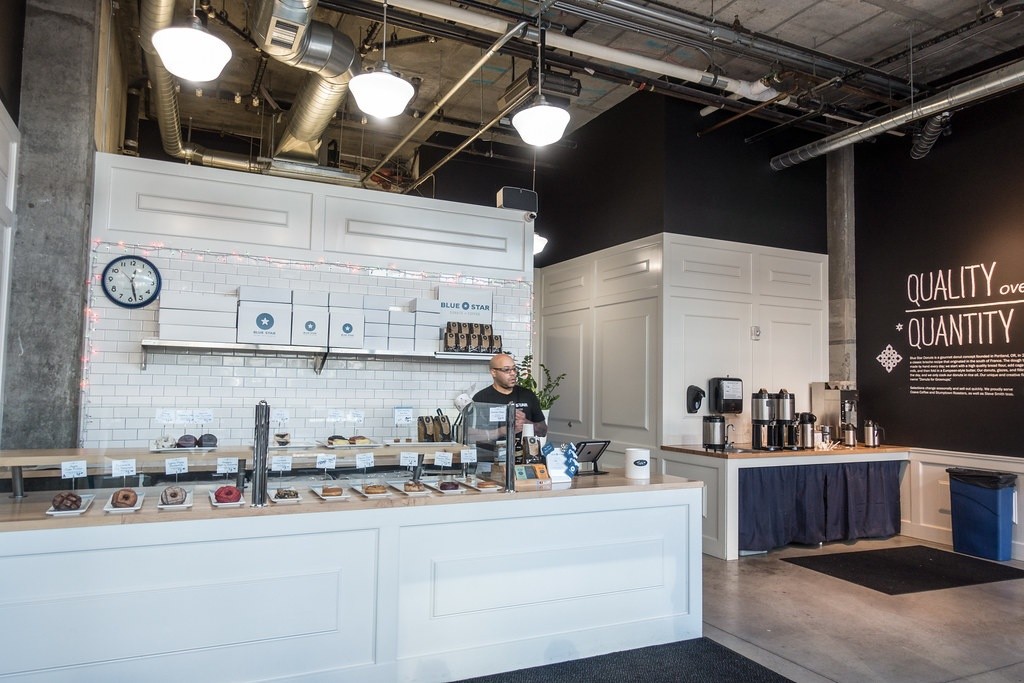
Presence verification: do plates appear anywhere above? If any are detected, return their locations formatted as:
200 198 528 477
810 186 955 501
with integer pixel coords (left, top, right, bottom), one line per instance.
209 488 245 507
349 483 392 497
316 440 382 449
456 478 502 491
308 484 350 500
104 491 145 512
384 438 455 446
386 482 431 495
46 494 96 515
424 480 466 493
249 442 316 449
267 487 302 503
151 445 219 453
158 487 193 509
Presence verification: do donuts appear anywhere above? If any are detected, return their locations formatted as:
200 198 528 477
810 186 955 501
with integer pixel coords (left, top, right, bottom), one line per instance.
111 488 138 508
439 481 459 490
274 490 298 499
161 486 186 505
214 486 241 503
53 492 83 510
320 486 344 496
155 433 218 449
364 484 386 494
403 484 425 492
476 482 496 488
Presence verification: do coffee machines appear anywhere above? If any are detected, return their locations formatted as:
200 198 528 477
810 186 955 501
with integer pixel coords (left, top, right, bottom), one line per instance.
752 389 797 449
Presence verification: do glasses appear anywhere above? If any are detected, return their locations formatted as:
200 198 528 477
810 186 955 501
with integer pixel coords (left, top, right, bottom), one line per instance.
493 367 518 373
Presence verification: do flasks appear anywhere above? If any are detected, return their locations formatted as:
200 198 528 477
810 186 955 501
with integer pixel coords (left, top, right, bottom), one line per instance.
798 412 816 449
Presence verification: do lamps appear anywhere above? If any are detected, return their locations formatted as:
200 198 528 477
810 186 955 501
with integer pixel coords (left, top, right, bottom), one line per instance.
508 14 570 148
347 2 415 120
149 0 233 82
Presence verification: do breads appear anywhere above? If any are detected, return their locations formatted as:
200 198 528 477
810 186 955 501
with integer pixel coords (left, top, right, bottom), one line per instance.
326 435 371 447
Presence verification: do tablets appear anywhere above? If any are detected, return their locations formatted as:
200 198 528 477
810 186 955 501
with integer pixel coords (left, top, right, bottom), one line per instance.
575 442 608 462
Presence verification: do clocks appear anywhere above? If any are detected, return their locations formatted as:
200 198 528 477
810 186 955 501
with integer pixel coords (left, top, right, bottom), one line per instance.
102 255 163 309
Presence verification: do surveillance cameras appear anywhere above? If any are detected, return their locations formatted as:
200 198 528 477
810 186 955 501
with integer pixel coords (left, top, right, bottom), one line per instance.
526 212 537 222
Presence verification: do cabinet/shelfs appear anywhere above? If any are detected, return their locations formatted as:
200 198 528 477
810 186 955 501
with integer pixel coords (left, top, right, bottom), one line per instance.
0 443 705 683
664 445 1024 575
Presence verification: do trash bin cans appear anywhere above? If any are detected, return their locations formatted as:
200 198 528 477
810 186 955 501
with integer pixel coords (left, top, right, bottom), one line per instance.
946 467 1018 562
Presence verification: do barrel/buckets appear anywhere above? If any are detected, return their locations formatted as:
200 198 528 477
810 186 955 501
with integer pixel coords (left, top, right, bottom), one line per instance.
625 448 650 479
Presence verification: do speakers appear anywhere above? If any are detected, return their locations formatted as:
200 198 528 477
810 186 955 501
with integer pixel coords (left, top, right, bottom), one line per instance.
496 186 538 213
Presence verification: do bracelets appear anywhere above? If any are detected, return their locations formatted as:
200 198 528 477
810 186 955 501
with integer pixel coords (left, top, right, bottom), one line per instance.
486 429 489 442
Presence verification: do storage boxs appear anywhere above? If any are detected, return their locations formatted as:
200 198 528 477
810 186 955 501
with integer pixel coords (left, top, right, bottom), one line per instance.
159 285 502 354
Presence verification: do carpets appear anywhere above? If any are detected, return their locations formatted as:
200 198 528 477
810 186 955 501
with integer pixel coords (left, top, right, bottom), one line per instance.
779 543 1024 595
454 636 797 683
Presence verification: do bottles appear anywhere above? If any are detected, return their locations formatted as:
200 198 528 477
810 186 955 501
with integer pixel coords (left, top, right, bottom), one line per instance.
515 438 523 465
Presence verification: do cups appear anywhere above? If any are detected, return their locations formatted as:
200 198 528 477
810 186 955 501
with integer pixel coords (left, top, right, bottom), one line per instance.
521 424 534 445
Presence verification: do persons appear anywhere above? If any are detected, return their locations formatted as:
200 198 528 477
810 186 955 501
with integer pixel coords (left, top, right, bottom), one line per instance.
453 355 548 478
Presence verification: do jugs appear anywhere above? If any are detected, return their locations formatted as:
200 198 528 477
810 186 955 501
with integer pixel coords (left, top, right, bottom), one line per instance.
864 419 885 447
844 423 858 447
